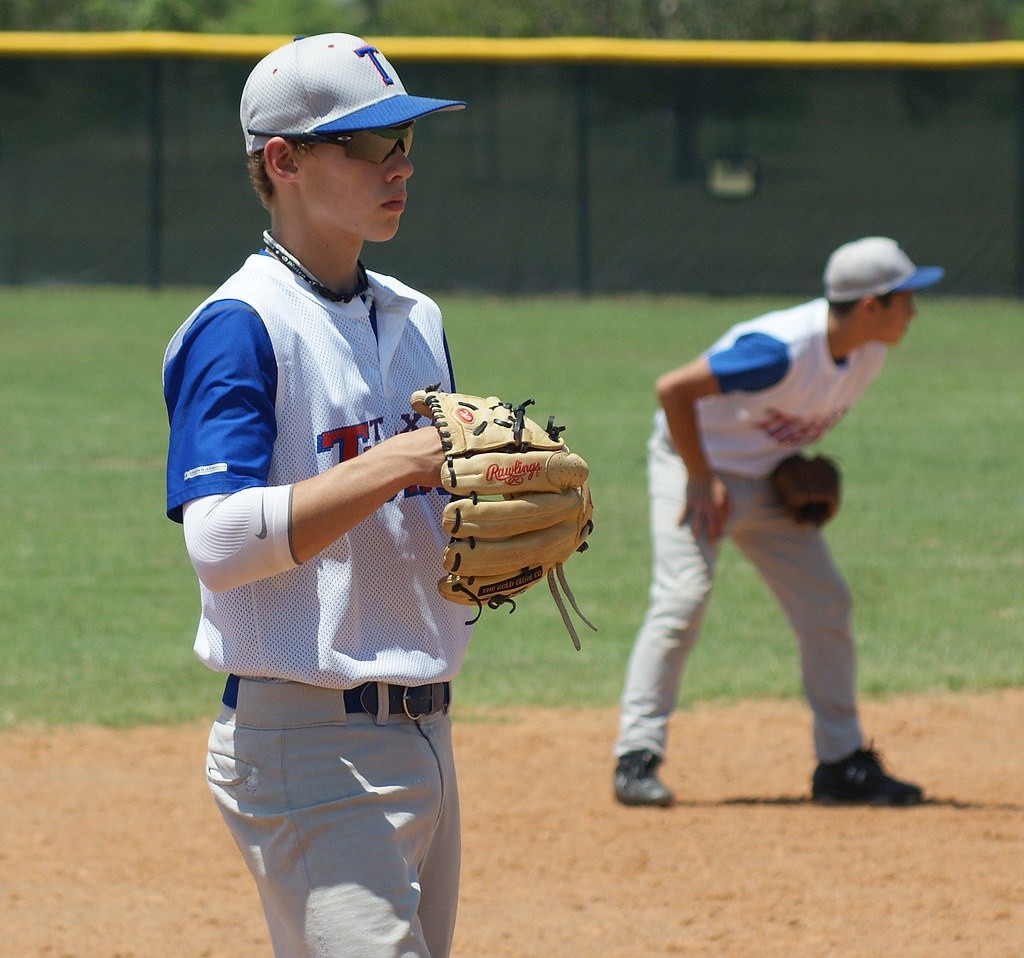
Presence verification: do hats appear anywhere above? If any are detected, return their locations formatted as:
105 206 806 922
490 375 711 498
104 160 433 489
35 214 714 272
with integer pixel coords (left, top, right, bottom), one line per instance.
240 32 468 156
824 236 946 303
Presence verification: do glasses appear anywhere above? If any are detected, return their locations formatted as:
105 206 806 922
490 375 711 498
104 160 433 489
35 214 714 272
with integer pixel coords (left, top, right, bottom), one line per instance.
248 120 416 164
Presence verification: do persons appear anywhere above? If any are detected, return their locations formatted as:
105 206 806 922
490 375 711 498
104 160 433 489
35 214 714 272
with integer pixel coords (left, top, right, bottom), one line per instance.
160 31 463 958
608 235 944 809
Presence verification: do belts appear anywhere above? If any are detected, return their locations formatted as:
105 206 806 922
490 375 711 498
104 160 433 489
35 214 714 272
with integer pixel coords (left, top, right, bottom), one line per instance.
222 673 452 720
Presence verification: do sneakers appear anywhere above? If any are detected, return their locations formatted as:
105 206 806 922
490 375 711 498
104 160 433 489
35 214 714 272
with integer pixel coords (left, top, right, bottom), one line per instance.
812 739 921 806
615 749 672 807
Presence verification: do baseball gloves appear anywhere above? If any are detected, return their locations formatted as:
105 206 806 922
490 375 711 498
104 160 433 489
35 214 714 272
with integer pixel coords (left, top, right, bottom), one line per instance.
407 382 600 613
766 450 842 531
